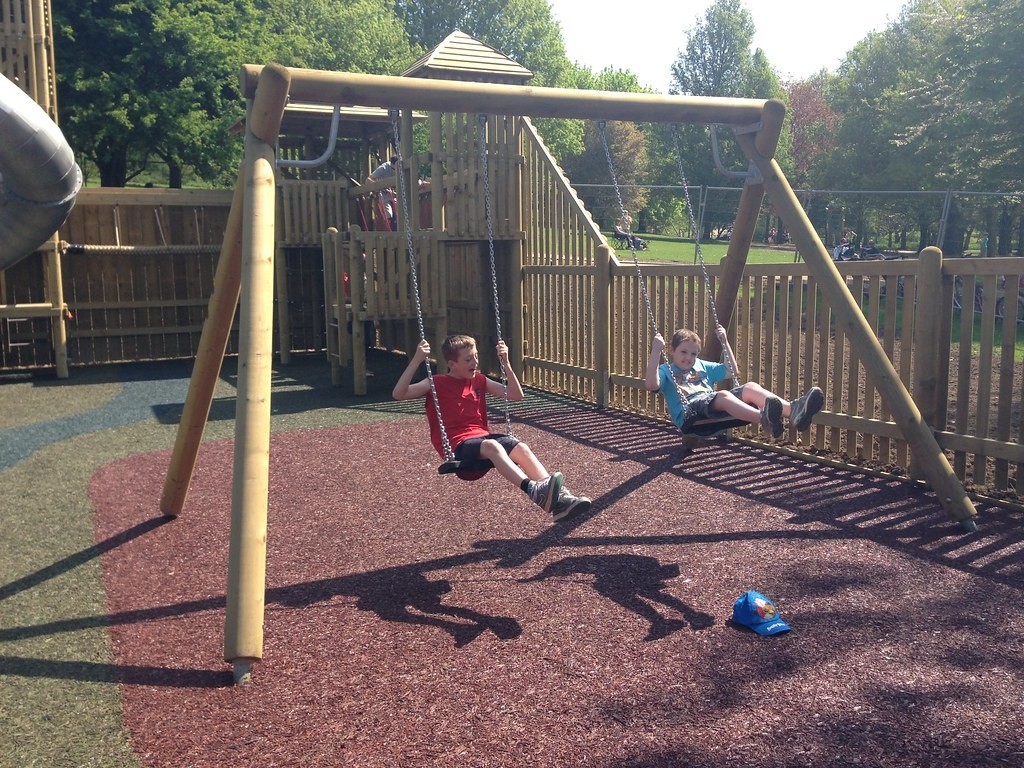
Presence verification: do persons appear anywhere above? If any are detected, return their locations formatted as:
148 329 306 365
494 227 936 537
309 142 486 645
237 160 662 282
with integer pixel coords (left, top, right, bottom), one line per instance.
615 218 634 249
768 228 775 242
782 232 789 243
862 240 882 259
347 254 379 364
834 238 858 259
632 236 646 250
728 226 733 237
365 156 431 230
978 233 988 256
393 336 591 522
619 211 632 249
646 324 824 438
956 254 983 310
1002 253 1024 288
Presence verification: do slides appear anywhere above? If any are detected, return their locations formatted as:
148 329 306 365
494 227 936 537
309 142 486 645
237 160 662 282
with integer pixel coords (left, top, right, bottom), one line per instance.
0 73 83 272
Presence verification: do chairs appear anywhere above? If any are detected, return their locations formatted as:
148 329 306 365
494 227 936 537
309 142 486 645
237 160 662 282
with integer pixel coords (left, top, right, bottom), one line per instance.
865 254 883 260
612 228 631 250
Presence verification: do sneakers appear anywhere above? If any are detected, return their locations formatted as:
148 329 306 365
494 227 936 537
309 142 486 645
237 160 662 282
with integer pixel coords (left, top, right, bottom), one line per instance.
533 472 563 513
552 486 591 522
760 397 785 438
788 386 824 432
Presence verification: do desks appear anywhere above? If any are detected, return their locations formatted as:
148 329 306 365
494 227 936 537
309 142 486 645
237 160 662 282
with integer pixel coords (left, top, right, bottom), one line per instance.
833 255 898 260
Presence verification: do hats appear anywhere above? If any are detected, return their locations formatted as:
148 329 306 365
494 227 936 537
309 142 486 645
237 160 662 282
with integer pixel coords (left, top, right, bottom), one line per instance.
733 591 791 636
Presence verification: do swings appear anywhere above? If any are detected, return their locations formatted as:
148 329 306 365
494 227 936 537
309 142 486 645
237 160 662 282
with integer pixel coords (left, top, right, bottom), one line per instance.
386 107 519 477
599 119 761 436
